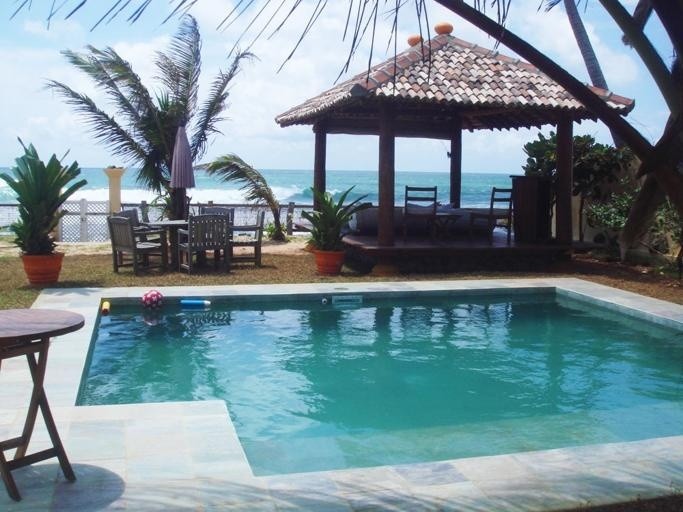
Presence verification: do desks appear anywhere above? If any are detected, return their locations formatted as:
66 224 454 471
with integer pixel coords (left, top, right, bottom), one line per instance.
0 308 86 501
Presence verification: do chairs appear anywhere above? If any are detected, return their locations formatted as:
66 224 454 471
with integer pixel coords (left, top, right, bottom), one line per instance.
106 207 265 274
468 186 518 245
402 185 438 244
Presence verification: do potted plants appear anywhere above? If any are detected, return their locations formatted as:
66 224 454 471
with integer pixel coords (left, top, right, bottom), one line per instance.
0 134 88 285
299 184 372 276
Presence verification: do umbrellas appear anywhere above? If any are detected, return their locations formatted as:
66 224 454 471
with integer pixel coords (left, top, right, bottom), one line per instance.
168 125 196 219
